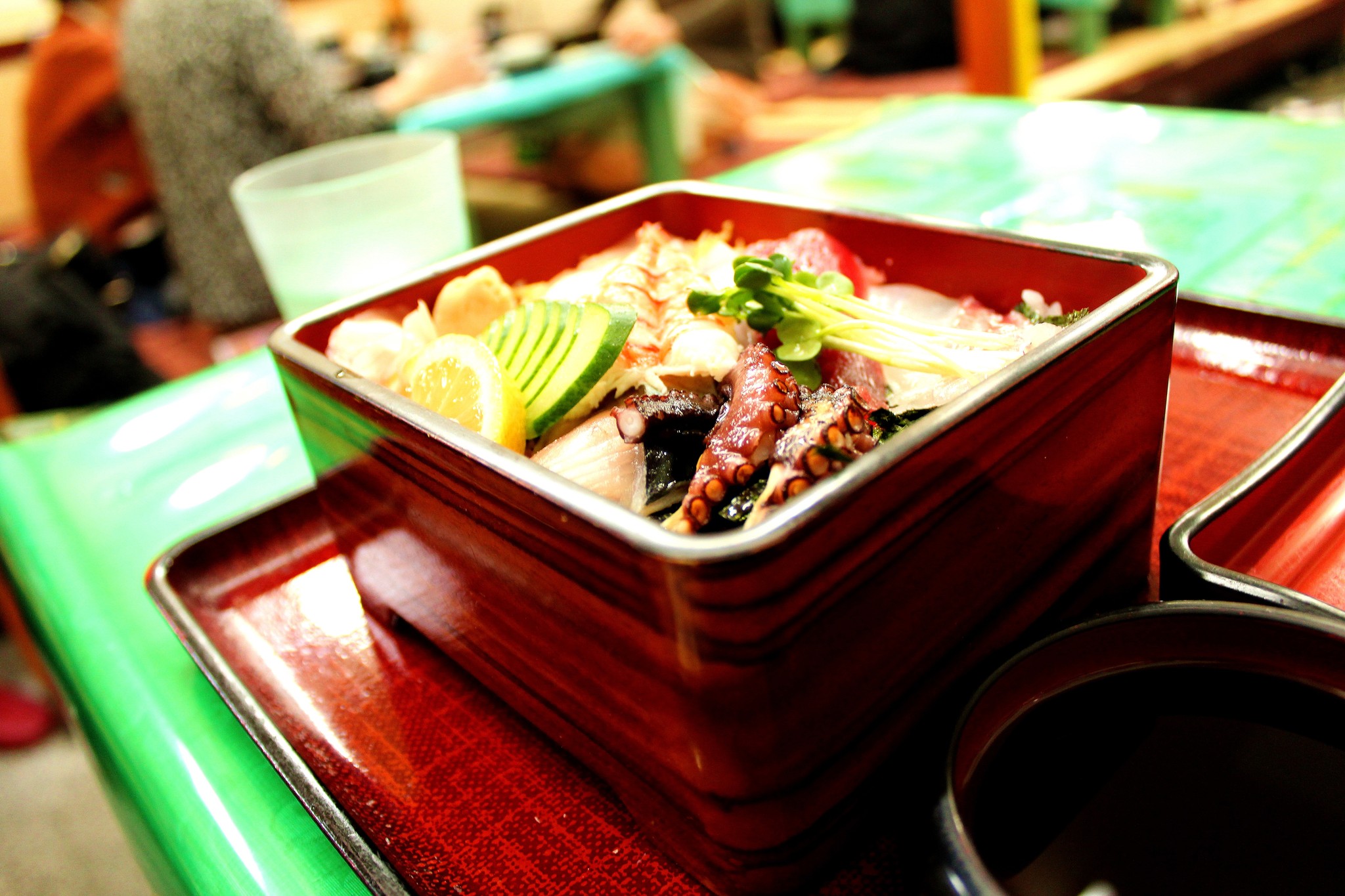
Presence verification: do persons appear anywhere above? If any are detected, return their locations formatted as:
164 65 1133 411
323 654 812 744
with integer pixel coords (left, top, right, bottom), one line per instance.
599 0 776 76
21 0 182 325
116 0 484 329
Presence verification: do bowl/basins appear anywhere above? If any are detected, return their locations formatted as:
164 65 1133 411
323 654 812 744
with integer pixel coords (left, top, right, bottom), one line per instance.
946 601 1345 896
1158 370 1345 623
497 33 551 78
264 180 1181 896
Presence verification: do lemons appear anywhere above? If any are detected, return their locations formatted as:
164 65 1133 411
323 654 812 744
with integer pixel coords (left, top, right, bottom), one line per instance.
385 332 526 456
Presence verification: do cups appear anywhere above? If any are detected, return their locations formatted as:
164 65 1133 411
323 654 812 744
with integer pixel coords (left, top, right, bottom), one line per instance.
229 128 472 323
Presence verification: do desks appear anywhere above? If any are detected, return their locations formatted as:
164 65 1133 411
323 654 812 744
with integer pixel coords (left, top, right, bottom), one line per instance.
0 94 1345 896
392 34 685 249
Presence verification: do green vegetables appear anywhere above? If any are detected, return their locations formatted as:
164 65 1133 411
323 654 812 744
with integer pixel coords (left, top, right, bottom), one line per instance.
686 253 1014 381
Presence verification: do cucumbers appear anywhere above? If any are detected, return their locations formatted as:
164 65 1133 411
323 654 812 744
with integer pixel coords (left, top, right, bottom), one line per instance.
469 294 638 438
